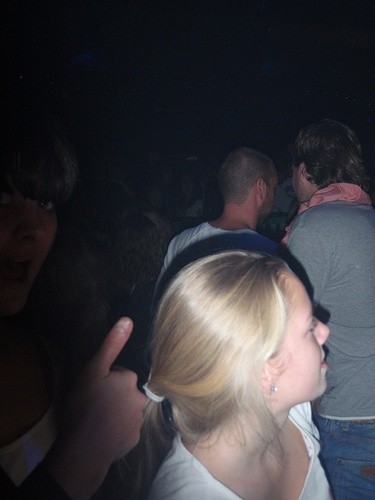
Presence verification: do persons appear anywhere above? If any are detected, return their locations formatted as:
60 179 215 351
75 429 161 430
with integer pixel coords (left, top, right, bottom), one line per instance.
29 116 375 500
0 93 148 500
